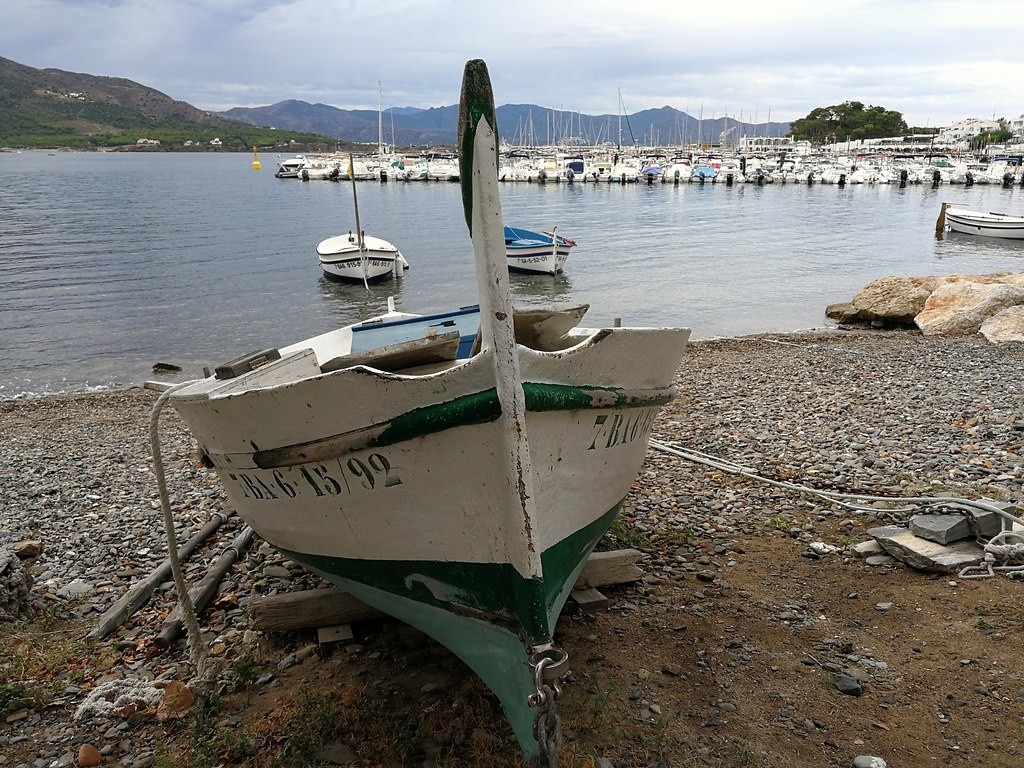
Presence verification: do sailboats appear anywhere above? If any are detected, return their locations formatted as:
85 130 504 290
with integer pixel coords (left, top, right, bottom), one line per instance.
274 84 1024 188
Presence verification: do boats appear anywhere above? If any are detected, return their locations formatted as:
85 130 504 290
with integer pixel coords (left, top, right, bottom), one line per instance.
503 225 578 276
316 151 409 280
944 205 1024 239
141 57 692 767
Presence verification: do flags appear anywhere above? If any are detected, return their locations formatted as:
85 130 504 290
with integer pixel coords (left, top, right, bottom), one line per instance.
347 155 352 179
